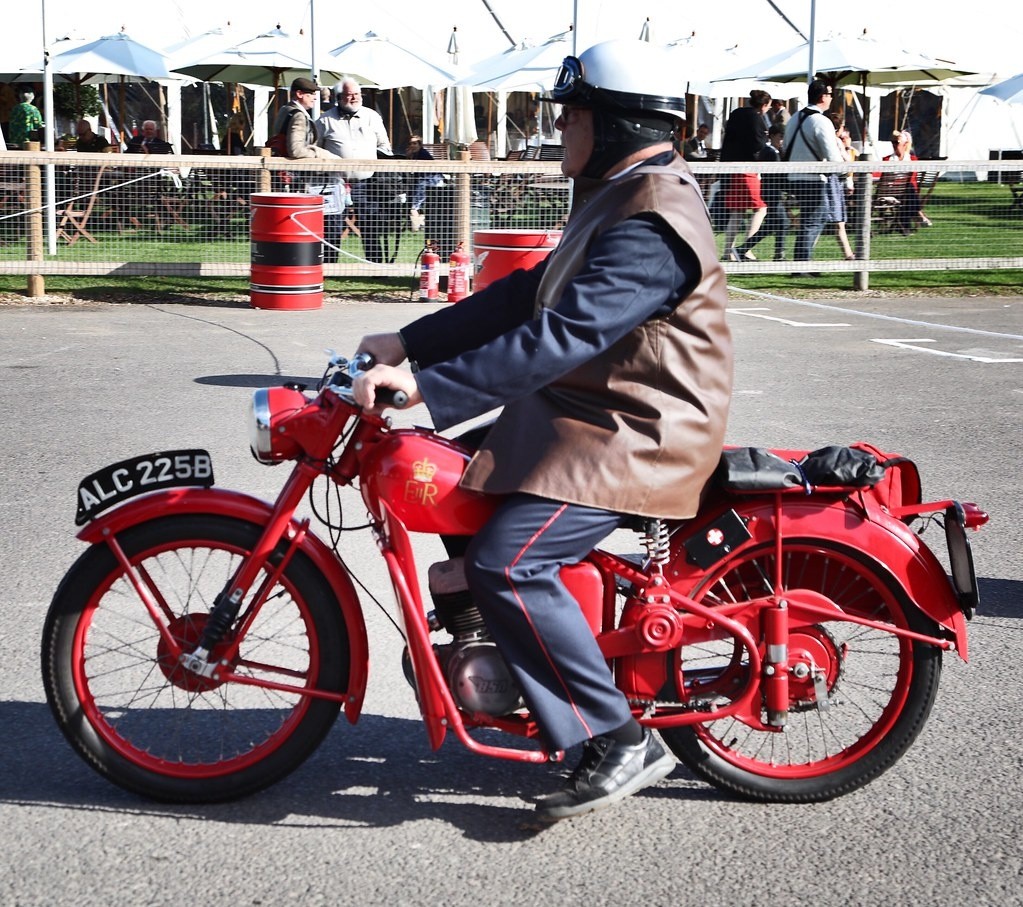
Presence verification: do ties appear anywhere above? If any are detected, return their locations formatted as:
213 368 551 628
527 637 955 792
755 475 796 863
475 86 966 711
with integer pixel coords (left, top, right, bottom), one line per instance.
699 142 705 155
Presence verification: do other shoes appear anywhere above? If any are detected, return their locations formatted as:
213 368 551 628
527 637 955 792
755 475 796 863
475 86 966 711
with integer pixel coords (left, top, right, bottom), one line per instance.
792 270 820 277
721 253 741 261
919 218 933 227
734 247 758 262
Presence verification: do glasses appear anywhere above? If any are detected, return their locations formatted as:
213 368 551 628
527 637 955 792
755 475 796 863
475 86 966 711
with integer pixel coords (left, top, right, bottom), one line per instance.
550 55 586 95
825 91 834 98
79 129 89 136
891 139 906 145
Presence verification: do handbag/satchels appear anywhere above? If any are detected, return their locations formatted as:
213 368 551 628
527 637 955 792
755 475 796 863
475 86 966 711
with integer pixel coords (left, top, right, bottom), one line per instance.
264 134 291 158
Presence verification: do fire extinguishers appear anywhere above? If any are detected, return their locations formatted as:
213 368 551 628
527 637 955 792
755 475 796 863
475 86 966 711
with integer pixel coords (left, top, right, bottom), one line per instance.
448 241 470 304
409 239 441 303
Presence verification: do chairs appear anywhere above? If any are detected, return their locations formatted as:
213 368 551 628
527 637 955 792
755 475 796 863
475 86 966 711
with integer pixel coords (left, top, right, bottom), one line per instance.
56 168 248 245
422 141 568 227
870 156 948 239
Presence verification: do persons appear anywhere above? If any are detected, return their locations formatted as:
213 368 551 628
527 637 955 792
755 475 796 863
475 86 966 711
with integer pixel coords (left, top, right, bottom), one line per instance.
125 120 175 154
406 135 449 227
351 39 735 817
474 103 526 128
8 88 46 149
272 78 394 262
75 120 112 152
673 79 932 276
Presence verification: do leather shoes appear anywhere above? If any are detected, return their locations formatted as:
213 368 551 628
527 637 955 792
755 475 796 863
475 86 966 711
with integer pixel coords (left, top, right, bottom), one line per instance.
535 727 675 820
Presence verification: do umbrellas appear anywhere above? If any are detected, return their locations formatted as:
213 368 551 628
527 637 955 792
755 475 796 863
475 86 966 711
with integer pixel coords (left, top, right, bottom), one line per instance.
0 23 1022 160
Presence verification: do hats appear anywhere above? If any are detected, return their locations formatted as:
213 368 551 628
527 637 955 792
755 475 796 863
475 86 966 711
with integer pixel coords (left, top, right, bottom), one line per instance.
291 76 323 93
768 123 787 135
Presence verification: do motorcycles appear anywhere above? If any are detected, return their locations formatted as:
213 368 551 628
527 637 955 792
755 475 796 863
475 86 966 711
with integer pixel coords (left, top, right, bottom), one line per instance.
39 357 989 807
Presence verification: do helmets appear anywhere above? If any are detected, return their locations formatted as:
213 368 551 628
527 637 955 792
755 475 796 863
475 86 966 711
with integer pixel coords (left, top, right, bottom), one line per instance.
554 36 689 121
